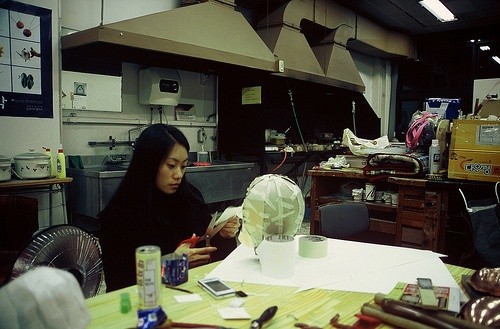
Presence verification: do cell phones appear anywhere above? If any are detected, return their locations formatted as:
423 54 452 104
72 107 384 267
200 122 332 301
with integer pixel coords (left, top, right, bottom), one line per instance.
198 278 235 298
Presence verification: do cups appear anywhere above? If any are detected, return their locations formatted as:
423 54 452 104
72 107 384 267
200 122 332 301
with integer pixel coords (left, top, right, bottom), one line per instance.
352 184 399 206
255 234 296 279
318 145 323 151
313 144 318 151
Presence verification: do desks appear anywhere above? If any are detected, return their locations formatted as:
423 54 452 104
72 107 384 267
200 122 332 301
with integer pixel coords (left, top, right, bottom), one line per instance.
61 232 486 329
0 177 73 226
305 168 500 253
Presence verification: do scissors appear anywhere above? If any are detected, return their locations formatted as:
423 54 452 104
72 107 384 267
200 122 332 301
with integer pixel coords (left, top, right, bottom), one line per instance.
179 211 233 268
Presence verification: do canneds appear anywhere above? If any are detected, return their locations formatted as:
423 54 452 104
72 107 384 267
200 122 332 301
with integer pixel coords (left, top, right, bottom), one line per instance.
135 245 161 306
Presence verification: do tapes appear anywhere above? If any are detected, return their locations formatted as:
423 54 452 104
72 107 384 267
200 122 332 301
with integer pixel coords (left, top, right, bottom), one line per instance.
299 235 327 258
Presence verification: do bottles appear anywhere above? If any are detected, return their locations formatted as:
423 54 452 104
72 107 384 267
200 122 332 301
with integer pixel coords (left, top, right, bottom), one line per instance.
56 148 66 179
42 146 56 176
428 139 440 174
473 97 479 116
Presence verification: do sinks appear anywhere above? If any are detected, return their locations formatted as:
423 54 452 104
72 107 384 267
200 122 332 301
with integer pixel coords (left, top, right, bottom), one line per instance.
83 166 127 172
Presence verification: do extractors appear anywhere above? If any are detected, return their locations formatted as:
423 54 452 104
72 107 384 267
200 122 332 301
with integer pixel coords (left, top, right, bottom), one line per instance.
59 0 285 72
255 22 365 94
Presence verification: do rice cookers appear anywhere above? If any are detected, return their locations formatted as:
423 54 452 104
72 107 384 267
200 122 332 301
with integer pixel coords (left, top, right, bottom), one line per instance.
0 154 15 181
13 148 51 179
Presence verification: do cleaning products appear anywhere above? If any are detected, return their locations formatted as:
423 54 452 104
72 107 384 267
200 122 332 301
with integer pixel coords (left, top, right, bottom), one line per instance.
57 147 66 178
42 146 54 177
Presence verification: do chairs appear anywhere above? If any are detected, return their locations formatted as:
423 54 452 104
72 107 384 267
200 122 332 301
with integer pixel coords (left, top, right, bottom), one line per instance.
318 204 385 245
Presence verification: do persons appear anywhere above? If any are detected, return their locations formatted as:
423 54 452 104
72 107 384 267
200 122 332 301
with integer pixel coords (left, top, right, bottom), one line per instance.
98 125 241 293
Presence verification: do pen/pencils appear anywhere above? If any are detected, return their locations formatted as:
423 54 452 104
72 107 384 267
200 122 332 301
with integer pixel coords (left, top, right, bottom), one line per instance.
166 286 193 294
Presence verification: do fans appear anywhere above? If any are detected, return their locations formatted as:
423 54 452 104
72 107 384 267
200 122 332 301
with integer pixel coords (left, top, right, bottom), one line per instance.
9 224 104 300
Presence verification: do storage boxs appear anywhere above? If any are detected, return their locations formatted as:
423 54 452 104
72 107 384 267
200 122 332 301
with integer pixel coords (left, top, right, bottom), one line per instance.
446 99 500 182
424 97 462 122
